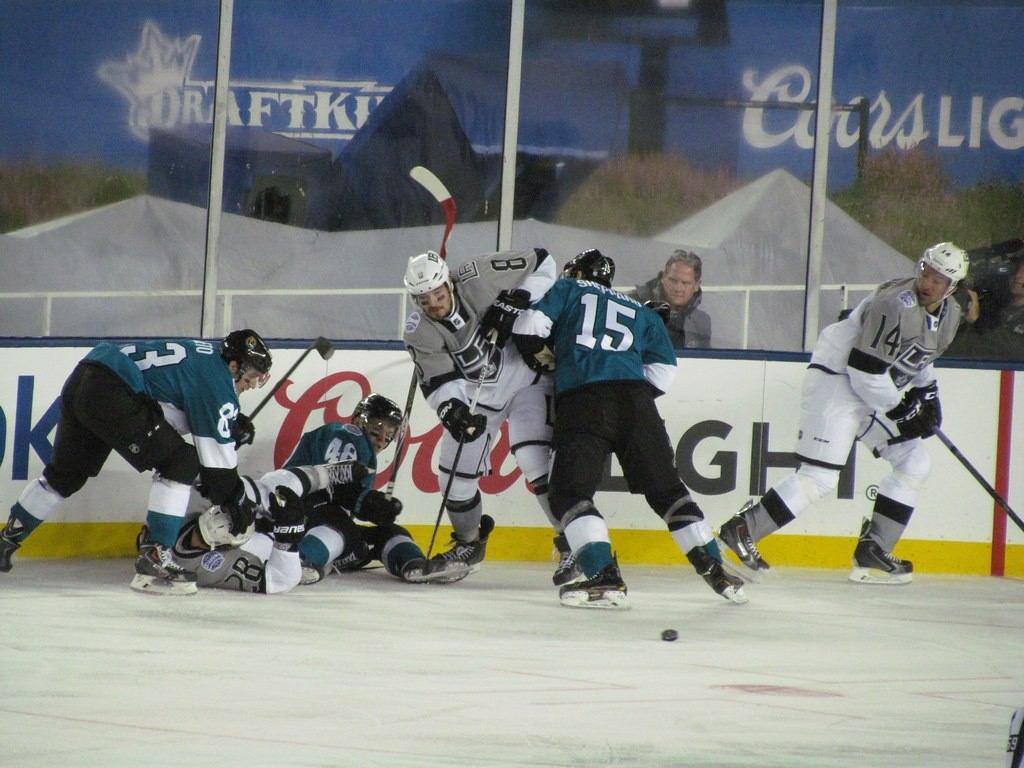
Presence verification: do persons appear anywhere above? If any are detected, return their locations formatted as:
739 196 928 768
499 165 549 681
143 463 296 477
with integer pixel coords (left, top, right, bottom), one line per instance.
175 391 474 593
623 249 712 348
718 242 969 586
0 329 273 597
510 249 751 609
402 247 586 588
938 251 1024 361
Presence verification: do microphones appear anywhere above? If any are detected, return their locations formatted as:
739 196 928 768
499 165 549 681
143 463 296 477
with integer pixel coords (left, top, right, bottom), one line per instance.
967 238 1023 260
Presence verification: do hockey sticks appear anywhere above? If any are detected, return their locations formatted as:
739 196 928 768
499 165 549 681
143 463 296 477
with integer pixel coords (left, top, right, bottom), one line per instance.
420 310 506 580
931 423 1024 532
244 335 336 427
384 166 458 502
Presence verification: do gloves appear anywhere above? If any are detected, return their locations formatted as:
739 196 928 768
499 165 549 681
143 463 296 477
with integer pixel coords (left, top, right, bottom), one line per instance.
220 479 261 537
231 413 256 451
885 390 928 440
479 288 537 345
436 398 487 444
357 490 402 526
269 485 305 544
909 379 944 439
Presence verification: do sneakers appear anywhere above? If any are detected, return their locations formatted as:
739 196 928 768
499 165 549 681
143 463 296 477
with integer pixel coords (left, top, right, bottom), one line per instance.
552 535 584 587
401 558 473 584
322 460 375 485
559 551 632 610
686 547 748 604
0 535 21 572
848 516 913 585
129 525 200 595
431 514 496 573
716 498 770 585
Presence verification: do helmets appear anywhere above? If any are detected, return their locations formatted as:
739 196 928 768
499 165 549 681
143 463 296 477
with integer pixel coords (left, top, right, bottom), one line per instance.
919 242 970 296
351 393 403 449
222 329 273 373
198 505 255 551
404 250 453 312
563 249 616 288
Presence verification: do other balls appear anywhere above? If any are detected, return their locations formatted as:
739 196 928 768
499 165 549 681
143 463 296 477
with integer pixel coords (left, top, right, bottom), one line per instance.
662 629 677 642
